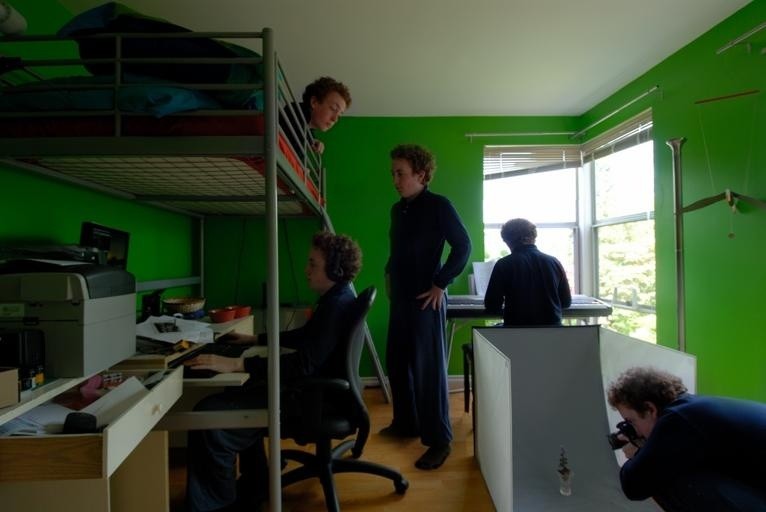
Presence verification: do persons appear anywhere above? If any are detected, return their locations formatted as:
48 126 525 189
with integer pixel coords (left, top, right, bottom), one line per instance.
484 219 573 327
278 75 352 169
604 366 765 511
184 232 360 511
382 142 471 469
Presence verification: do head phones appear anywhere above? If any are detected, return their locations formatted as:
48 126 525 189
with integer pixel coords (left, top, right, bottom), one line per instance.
327 234 350 280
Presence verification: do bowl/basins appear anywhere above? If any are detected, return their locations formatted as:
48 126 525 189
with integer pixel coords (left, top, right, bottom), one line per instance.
208 306 252 323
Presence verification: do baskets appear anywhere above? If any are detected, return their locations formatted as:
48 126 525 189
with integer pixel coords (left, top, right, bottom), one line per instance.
161 296 206 312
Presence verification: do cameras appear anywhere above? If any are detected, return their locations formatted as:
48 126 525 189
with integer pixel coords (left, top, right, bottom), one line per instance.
606 420 639 450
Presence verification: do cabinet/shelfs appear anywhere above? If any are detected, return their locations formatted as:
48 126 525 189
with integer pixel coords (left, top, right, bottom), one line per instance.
0 363 184 510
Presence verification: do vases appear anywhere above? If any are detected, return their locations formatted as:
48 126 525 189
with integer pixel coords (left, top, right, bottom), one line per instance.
557 470 571 497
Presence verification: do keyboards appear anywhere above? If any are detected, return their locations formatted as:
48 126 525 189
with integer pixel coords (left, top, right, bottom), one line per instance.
168 343 245 380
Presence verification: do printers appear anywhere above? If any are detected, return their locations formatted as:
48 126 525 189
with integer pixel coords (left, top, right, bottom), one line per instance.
0 244 137 377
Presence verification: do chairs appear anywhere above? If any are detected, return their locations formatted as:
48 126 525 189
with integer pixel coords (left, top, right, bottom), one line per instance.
279 284 409 511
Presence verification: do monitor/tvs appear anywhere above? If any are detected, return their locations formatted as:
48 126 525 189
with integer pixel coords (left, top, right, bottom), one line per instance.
80 221 129 274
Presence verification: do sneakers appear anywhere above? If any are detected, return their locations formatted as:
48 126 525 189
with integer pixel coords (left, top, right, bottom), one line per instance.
378 418 421 439
415 443 451 471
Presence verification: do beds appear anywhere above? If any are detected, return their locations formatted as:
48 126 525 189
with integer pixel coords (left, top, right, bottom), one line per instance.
0 16 325 217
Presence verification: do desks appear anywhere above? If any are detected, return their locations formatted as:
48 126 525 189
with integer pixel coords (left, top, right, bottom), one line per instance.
444 296 612 371
110 313 269 512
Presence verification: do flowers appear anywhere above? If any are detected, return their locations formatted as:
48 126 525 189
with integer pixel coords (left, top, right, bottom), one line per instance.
556 445 571 480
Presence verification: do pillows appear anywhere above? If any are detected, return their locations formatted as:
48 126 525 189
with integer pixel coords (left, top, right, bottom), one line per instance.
1 1 280 119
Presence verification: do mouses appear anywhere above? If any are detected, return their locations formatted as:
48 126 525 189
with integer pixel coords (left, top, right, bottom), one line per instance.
219 335 246 347
